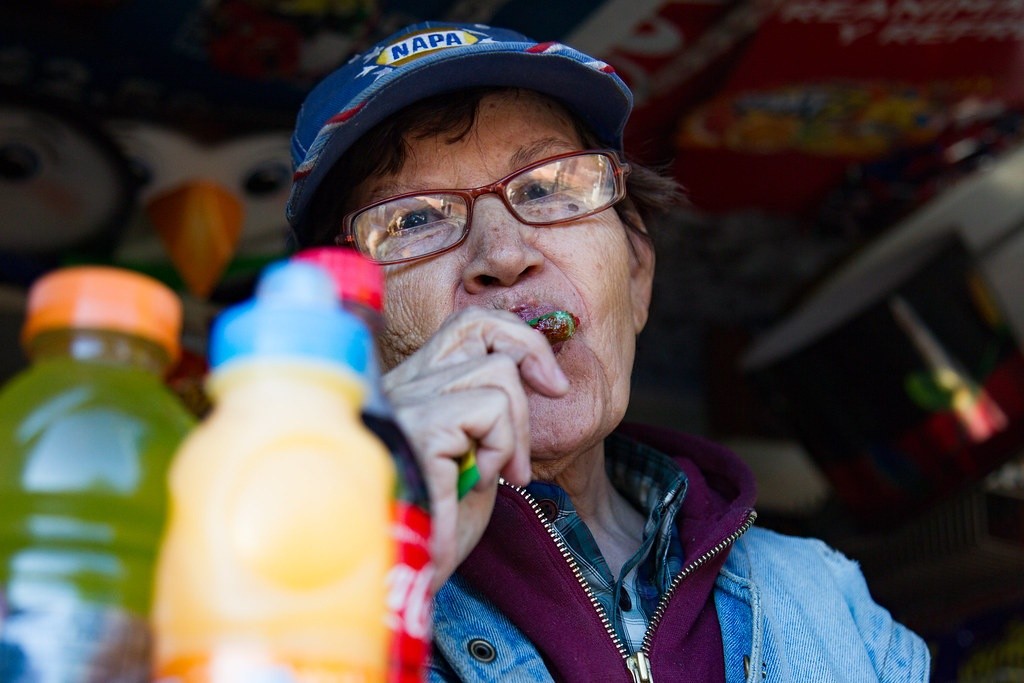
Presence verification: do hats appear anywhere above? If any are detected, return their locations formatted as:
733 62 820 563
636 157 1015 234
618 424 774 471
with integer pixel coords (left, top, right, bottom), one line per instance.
285 20 634 240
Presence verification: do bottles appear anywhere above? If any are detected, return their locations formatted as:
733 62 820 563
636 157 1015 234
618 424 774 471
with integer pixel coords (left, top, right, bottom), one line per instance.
154 262 396 683
0 265 181 683
887 293 1006 480
292 250 432 683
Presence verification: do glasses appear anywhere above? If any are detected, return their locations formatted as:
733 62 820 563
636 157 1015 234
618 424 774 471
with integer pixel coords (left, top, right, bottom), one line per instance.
335 149 632 267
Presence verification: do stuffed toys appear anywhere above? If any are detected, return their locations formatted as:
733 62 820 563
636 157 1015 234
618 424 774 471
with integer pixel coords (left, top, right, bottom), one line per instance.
0 46 304 339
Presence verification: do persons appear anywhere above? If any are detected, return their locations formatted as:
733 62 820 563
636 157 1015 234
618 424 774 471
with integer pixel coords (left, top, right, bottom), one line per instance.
284 21 932 683
